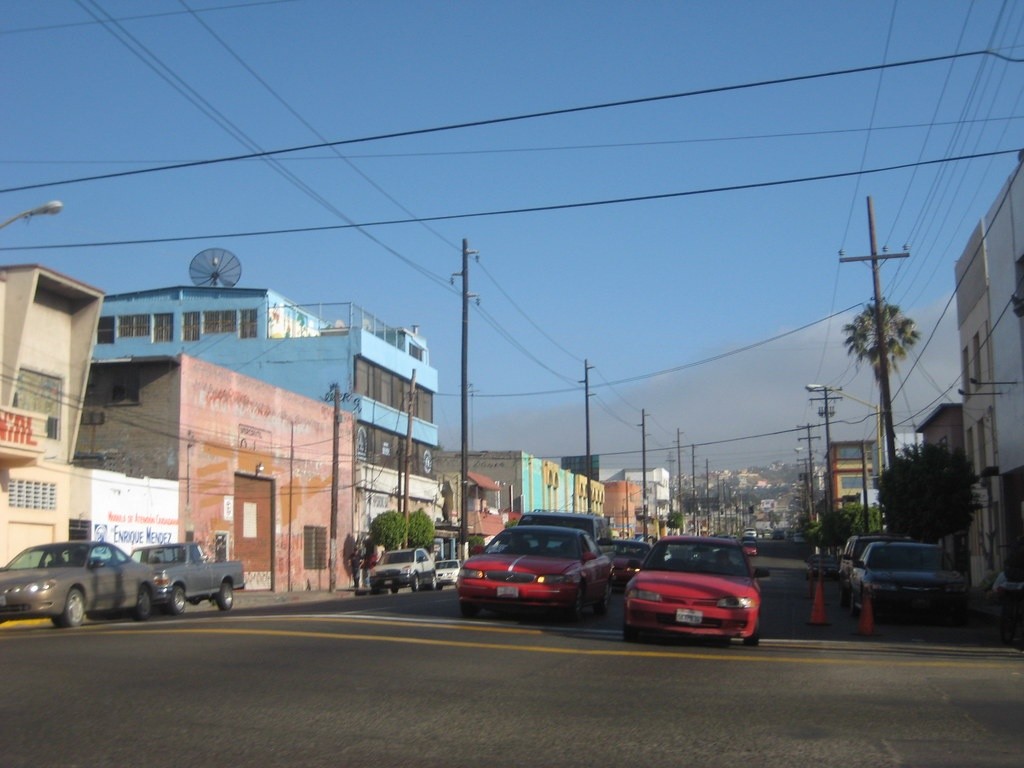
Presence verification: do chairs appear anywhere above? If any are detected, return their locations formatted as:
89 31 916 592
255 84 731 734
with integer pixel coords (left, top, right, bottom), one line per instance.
47 550 87 566
518 540 578 555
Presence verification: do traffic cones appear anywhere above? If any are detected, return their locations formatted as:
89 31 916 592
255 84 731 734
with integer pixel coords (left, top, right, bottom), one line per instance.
852 585 883 637
806 582 833 627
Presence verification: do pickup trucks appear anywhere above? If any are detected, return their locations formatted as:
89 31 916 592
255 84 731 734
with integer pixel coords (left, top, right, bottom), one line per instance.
127 542 248 616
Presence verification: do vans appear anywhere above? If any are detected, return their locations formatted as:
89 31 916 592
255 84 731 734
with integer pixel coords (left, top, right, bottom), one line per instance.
513 510 616 569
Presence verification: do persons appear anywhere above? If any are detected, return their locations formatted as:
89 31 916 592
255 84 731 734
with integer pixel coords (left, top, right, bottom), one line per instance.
348 548 364 588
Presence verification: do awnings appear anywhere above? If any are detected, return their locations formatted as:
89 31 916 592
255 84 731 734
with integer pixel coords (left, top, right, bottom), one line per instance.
467 473 501 491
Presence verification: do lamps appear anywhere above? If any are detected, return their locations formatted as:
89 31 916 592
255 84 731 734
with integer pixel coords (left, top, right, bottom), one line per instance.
256 462 265 477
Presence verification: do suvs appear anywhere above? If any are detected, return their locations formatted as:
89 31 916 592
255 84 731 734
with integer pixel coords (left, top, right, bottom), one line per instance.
369 546 437 593
835 533 916 610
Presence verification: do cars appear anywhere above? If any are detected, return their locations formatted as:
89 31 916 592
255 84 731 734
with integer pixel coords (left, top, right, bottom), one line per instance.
610 538 655 589
713 528 785 557
620 534 771 648
846 540 970 629
452 524 616 622
435 559 462 590
803 553 838 580
793 532 808 544
0 541 155 630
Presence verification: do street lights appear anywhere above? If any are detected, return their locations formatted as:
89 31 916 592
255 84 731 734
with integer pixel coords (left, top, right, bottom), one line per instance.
804 384 883 534
